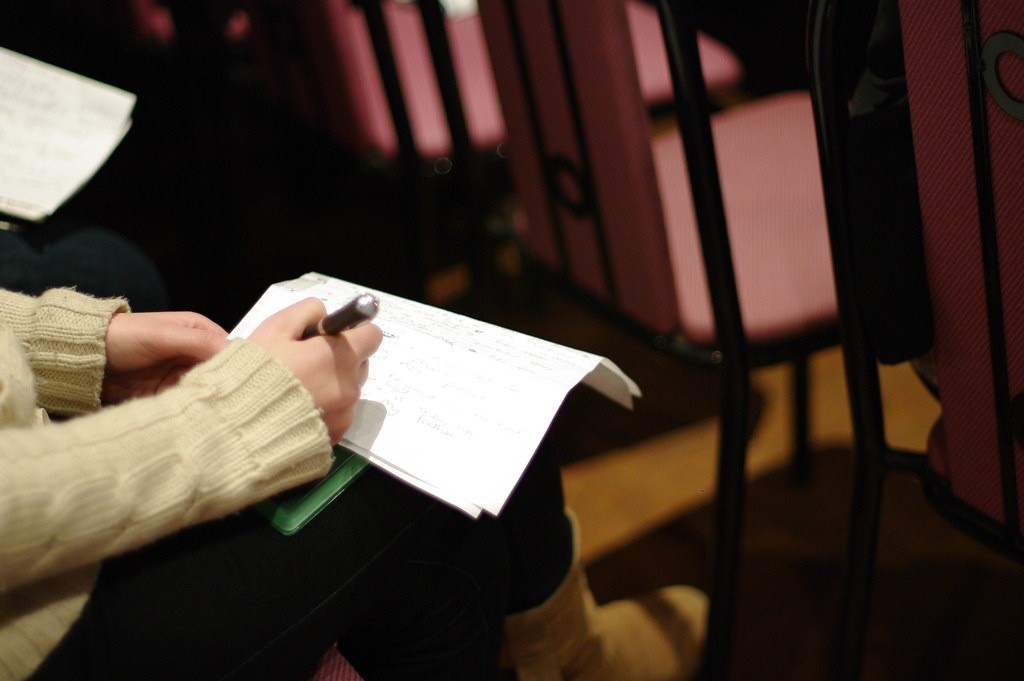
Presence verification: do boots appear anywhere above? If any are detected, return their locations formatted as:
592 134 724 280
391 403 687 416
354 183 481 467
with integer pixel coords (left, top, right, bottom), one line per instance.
504 502 710 681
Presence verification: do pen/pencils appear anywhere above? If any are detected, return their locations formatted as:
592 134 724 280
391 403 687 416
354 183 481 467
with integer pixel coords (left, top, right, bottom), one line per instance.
302 294 379 340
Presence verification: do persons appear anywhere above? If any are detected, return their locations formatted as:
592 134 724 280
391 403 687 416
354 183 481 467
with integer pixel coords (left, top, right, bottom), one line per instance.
1 287 707 681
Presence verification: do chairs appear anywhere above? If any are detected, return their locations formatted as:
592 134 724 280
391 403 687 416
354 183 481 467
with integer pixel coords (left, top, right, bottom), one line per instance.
0 0 1024 681
